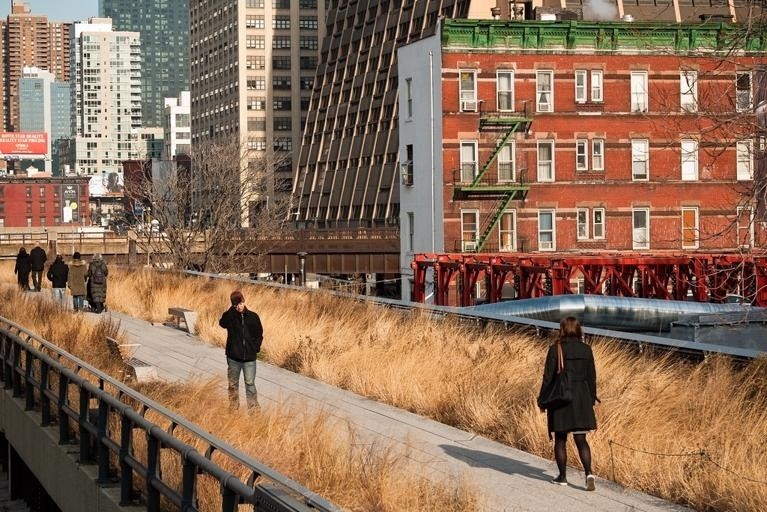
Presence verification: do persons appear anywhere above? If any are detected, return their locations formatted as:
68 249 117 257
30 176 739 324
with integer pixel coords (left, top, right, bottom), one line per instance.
218 290 263 417
14 246 32 291
28 240 46 292
88 250 109 314
536 315 601 491
68 251 89 312
46 254 69 307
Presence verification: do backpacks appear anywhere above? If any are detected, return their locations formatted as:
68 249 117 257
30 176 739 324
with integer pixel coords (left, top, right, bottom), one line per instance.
91 263 105 284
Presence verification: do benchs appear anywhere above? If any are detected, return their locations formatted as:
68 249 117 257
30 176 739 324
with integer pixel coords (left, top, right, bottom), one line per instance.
168 307 199 334
105 337 156 384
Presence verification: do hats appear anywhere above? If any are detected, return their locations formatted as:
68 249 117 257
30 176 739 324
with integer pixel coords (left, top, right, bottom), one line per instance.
55 250 102 260
229 291 245 305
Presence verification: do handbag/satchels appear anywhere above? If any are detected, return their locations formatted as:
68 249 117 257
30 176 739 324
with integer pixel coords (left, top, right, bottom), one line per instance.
537 371 572 409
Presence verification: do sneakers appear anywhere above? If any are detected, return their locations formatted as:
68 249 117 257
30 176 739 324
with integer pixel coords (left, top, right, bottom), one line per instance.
585 474 597 491
550 477 569 485
